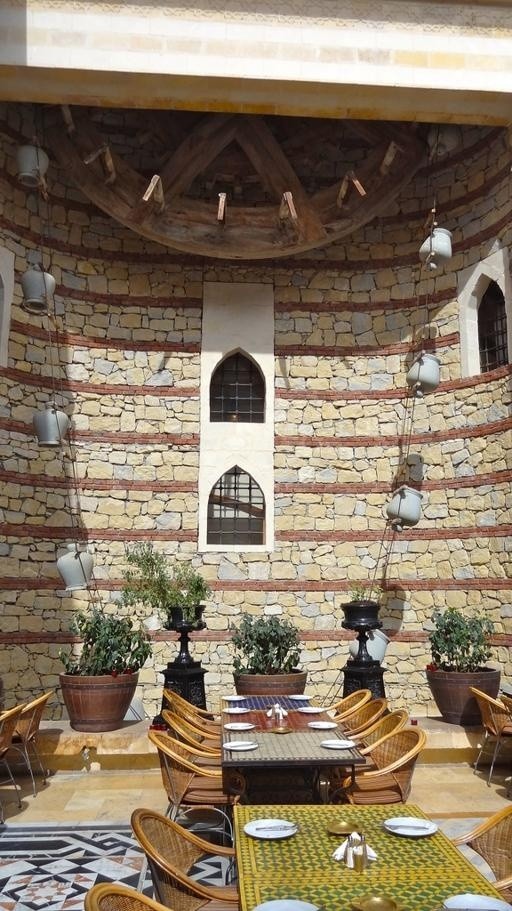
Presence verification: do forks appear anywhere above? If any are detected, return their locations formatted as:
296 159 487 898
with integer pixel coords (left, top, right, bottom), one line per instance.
386 825 428 831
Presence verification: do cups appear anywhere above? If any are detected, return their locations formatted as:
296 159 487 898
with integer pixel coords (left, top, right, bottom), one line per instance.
264 704 291 735
342 836 373 872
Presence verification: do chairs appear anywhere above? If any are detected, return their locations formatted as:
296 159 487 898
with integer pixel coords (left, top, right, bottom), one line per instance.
11 689 57 796
0 701 25 813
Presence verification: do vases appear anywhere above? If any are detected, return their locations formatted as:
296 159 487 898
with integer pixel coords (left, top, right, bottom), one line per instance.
419 227 455 266
385 487 425 529
56 541 95 592
15 132 51 182
16 257 57 311
405 351 442 393
32 396 71 447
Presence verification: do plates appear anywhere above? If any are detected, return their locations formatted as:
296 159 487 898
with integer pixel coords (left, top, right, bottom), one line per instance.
253 899 319 911
438 895 510 911
288 694 338 730
319 739 355 751
348 894 401 911
325 822 362 836
383 816 439 839
221 695 259 752
243 818 298 840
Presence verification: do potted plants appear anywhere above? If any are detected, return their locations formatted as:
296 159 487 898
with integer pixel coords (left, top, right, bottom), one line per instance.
232 616 307 696
58 612 152 733
421 606 502 722
336 574 382 627
119 543 205 627
